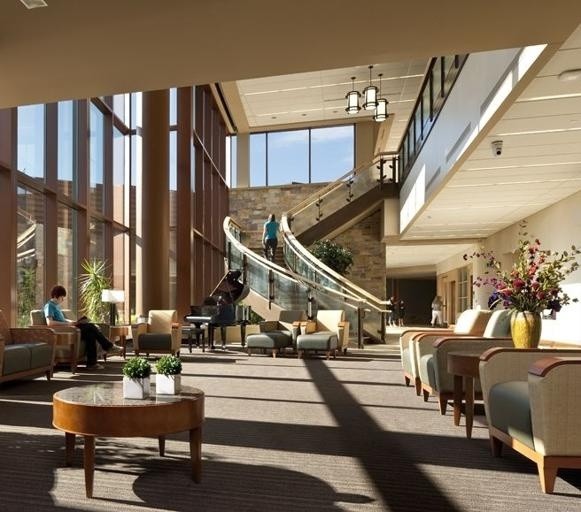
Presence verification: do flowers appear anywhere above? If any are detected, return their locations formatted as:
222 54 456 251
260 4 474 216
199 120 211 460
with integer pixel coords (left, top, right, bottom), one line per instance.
461 216 581 320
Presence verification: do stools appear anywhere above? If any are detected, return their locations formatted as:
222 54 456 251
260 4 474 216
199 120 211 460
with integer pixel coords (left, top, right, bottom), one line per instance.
179 325 206 354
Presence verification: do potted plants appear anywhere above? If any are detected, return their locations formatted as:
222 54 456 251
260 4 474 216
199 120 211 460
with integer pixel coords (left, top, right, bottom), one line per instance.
121 354 183 401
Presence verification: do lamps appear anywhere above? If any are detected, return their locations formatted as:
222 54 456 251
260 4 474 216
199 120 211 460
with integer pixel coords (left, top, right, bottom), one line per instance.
344 65 390 124
102 289 126 326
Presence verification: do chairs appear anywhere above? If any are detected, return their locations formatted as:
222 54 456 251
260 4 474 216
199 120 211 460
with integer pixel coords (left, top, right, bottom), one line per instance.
130 310 183 358
397 308 581 493
244 307 351 362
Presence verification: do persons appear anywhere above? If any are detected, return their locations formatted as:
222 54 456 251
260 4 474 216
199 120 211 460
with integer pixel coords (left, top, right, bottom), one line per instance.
44 285 124 372
262 213 284 262
395 299 408 326
386 293 396 327
429 296 446 328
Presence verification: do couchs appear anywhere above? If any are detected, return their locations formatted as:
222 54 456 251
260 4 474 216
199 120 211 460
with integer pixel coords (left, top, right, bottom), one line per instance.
0 309 112 382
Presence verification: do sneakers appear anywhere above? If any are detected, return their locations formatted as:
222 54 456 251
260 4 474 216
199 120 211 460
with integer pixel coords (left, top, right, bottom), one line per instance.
87 363 105 370
107 344 125 356
